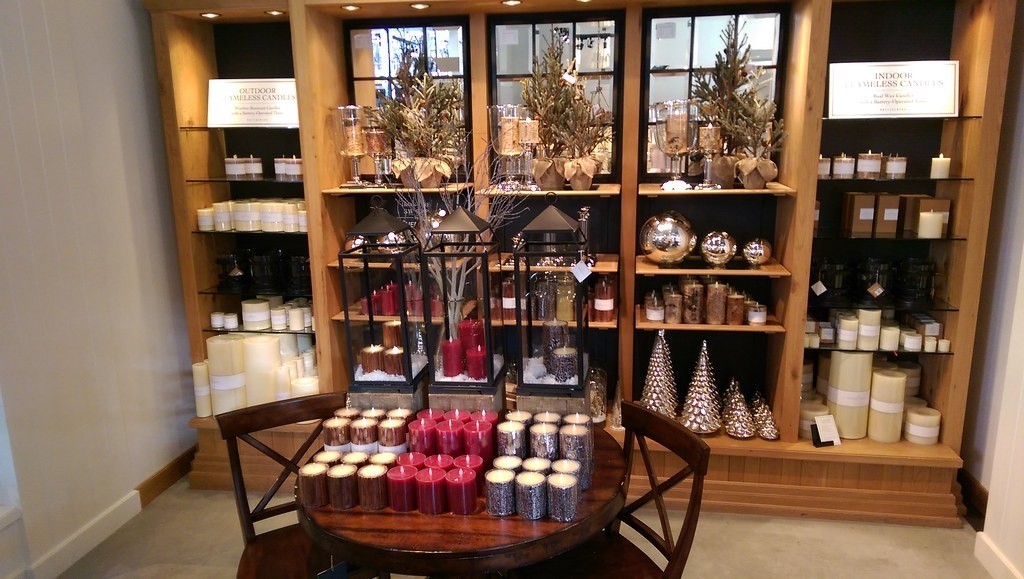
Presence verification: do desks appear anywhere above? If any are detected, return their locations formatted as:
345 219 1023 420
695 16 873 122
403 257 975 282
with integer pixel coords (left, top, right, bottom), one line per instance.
293 420 631 579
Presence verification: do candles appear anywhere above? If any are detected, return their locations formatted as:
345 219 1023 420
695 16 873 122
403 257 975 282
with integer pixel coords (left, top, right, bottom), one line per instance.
285 316 602 527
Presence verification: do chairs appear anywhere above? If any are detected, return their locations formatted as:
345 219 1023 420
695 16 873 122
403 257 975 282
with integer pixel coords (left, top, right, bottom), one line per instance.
510 395 710 579
212 393 346 578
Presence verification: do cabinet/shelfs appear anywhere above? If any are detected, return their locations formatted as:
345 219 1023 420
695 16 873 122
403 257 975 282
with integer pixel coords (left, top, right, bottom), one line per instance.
469 5 632 429
297 4 483 427
623 2 815 449
792 0 1017 450
151 2 326 432
